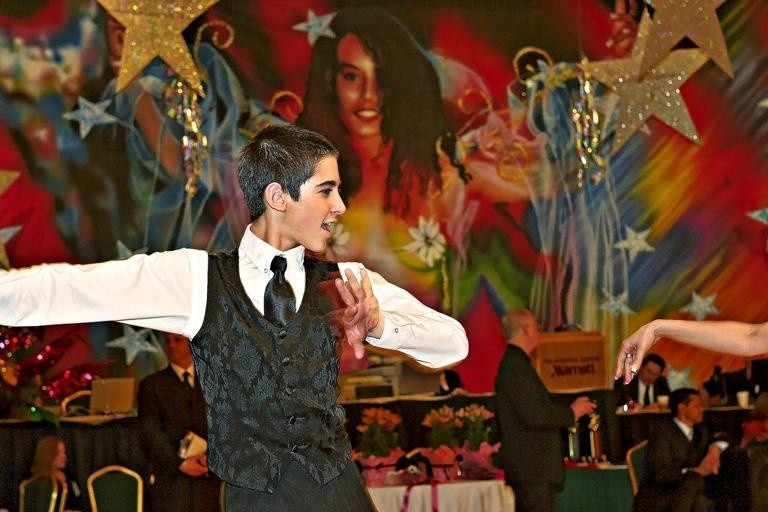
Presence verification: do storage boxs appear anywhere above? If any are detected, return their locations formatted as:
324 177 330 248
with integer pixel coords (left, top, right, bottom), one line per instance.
529 331 607 389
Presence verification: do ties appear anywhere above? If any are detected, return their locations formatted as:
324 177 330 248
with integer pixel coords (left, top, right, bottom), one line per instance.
181 373 193 403
645 384 650 408
263 255 295 328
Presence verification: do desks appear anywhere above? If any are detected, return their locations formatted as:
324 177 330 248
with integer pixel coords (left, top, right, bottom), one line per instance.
367 479 518 512
1 388 615 512
614 402 757 465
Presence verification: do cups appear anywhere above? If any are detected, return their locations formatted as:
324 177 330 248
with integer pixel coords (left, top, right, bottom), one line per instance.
736 392 749 408
656 395 669 413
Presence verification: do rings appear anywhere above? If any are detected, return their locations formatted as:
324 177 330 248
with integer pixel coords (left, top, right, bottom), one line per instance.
625 352 633 359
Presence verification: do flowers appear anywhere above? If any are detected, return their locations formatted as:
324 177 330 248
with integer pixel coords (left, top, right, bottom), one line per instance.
355 401 496 458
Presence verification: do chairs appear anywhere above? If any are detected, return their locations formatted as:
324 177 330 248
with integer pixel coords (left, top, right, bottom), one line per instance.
625 440 647 495
17 465 144 511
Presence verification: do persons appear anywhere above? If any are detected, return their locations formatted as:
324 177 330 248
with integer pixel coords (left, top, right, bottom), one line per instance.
611 316 768 387
24 435 83 510
136 330 219 511
494 308 599 511
1 124 474 511
613 352 672 410
104 7 559 313
631 386 732 511
